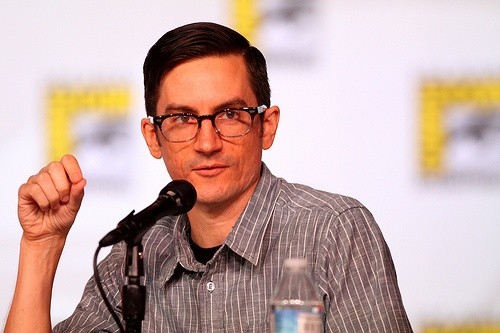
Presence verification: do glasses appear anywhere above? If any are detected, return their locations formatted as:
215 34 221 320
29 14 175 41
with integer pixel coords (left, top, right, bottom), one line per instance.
147 105 267 143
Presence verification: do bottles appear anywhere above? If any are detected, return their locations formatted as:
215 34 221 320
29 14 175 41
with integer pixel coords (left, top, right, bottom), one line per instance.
271 258 324 333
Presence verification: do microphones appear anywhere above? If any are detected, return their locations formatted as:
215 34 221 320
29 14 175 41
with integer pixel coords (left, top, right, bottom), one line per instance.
99 179 197 248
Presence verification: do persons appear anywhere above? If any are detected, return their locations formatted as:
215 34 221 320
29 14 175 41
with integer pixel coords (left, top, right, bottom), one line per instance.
2 22 412 333
